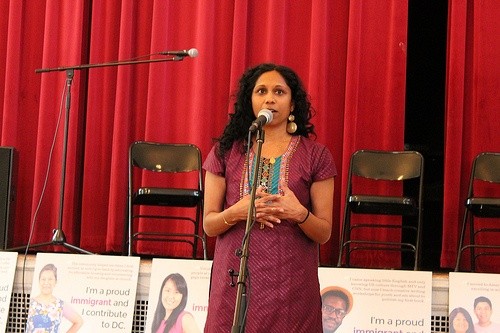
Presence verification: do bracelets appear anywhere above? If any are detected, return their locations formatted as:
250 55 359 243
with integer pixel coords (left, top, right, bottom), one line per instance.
297 210 310 225
219 210 238 225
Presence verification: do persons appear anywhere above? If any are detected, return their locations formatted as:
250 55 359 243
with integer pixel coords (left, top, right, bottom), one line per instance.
318 286 353 333
449 306 480 333
150 274 200 333
25 263 85 333
199 62 338 333
473 296 500 333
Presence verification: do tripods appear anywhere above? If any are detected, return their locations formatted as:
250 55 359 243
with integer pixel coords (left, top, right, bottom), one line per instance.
0 55 183 257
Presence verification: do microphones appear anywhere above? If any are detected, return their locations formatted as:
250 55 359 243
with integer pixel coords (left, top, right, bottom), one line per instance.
161 48 198 58
248 108 273 133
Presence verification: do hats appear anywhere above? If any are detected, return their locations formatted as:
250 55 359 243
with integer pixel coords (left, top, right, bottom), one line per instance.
320 286 353 315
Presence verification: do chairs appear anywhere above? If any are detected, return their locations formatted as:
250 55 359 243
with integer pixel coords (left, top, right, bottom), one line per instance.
122 142 207 261
337 149 425 271
454 152 500 272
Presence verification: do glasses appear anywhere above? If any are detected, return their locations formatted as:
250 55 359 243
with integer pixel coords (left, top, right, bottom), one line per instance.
322 305 346 319
